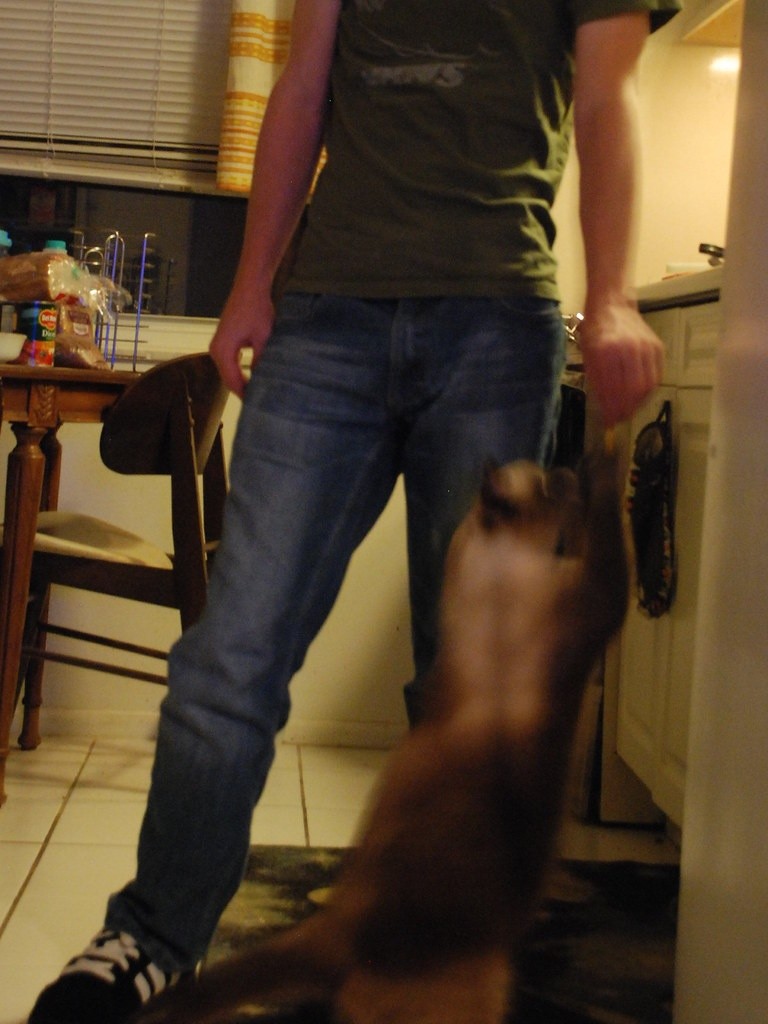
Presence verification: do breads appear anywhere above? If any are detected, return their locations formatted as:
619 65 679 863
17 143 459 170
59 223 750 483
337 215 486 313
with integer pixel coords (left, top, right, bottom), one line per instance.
0 251 110 370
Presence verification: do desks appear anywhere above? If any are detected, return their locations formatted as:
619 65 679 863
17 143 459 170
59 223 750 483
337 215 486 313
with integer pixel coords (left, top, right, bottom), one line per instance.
0 363 144 809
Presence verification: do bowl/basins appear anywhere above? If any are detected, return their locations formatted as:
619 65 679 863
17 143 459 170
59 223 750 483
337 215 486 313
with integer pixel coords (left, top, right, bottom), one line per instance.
0 332 27 364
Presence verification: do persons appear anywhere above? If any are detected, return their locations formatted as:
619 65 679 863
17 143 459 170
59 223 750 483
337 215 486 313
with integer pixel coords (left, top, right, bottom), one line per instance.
29 1 685 1024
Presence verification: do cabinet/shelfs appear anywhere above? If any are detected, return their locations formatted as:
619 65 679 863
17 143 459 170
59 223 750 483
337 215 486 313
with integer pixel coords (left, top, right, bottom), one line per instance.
596 299 716 829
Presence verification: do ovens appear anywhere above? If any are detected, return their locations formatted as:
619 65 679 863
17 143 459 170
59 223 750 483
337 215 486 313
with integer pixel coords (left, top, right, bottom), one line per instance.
551 369 604 477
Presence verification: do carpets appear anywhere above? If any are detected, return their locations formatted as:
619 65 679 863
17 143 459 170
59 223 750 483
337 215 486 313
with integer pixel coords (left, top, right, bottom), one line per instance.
198 844 679 1024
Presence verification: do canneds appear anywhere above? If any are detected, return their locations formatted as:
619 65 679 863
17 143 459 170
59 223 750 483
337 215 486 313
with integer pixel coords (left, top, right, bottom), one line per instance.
14 300 56 366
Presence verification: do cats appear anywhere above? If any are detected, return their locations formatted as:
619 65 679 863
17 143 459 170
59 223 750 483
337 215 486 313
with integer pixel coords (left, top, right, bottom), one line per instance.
131 429 628 1024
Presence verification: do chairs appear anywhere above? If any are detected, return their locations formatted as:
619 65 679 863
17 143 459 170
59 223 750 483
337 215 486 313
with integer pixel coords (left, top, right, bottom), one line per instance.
1 353 228 751
